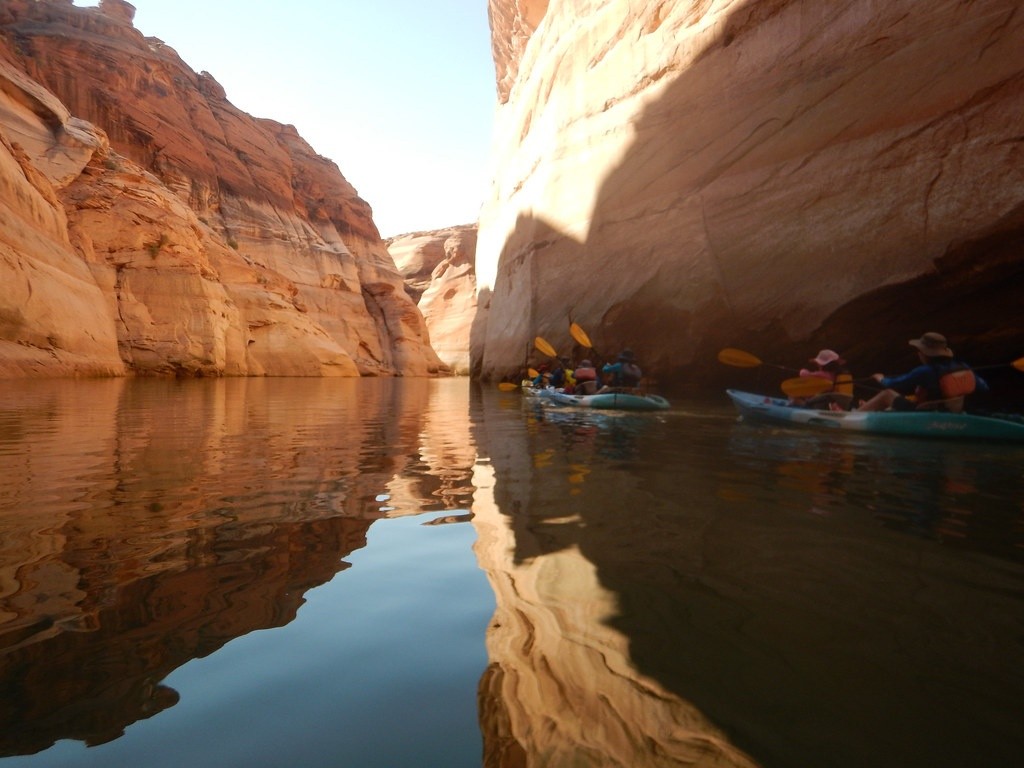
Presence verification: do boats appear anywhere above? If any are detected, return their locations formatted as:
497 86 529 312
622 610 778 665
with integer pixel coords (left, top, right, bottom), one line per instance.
725 389 1024 438
548 389 672 411
522 385 563 396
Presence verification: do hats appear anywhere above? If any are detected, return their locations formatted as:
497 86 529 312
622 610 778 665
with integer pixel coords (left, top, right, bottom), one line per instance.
561 356 570 363
537 365 547 372
814 349 839 366
616 348 638 363
908 333 954 358
576 359 591 368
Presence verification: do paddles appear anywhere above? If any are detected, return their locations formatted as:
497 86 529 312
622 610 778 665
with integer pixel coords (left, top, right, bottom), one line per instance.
718 349 881 393
569 322 607 366
781 357 1024 398
528 369 546 380
499 382 534 392
535 336 563 369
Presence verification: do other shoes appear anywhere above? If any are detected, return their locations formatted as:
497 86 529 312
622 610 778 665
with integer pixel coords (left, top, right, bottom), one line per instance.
859 399 865 406
762 399 771 404
593 388 598 394
829 402 841 411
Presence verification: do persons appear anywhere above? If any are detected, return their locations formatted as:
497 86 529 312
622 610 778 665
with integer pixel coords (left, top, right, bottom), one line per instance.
569 359 602 391
831 333 986 411
789 349 847 407
598 349 641 392
533 364 550 385
548 357 572 388
